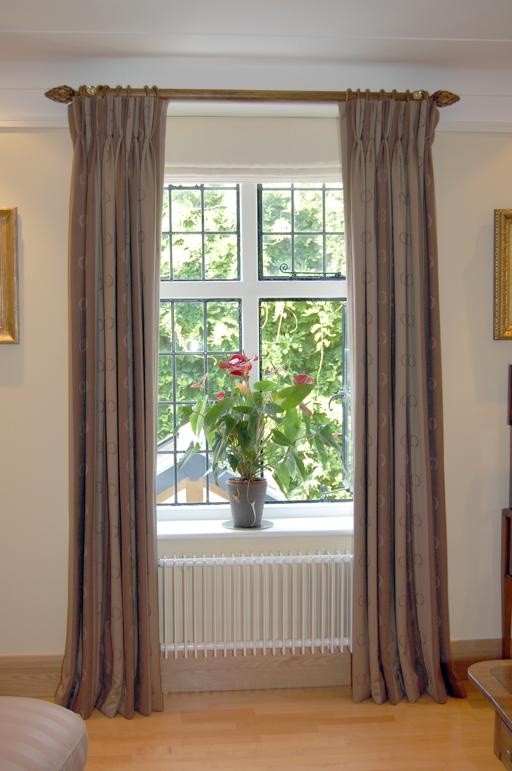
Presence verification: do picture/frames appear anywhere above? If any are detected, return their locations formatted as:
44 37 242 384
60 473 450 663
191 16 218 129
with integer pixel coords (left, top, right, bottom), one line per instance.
493 208 512 341
0 209 17 343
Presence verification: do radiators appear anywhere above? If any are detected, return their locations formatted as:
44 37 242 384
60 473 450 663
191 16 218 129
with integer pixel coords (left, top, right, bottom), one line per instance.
156 553 353 659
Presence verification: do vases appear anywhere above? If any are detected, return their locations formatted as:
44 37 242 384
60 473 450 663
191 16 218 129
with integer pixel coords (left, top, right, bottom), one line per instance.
227 479 267 527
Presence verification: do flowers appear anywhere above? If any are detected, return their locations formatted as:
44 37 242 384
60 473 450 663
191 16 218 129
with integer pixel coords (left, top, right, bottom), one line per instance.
178 354 337 501
